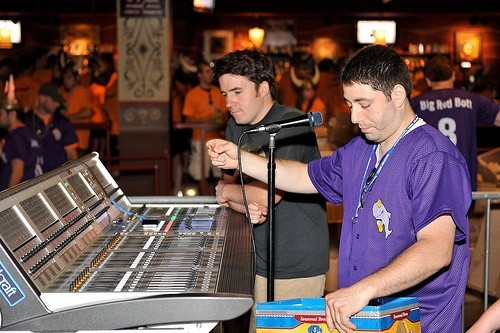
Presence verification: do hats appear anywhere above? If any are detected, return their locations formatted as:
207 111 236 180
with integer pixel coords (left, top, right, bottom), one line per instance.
41 84 64 102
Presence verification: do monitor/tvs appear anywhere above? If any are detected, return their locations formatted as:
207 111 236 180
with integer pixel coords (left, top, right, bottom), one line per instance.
354 18 400 47
0 17 26 49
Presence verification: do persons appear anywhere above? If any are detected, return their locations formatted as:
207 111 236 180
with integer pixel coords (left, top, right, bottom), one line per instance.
411 57 500 216
0 83 80 198
465 296 500 333
213 48 331 333
205 44 473 333
182 60 227 193
0 50 420 195
294 80 326 125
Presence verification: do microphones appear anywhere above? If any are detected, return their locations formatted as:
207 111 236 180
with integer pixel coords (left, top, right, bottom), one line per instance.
244 112 323 134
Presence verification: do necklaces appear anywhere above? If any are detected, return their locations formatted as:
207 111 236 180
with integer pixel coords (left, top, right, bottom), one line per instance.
352 114 419 225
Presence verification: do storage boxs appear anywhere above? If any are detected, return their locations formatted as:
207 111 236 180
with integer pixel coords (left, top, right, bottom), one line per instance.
255 297 421 333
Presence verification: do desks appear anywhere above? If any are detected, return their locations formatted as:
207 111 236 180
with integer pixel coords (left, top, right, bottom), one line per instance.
71 118 112 166
